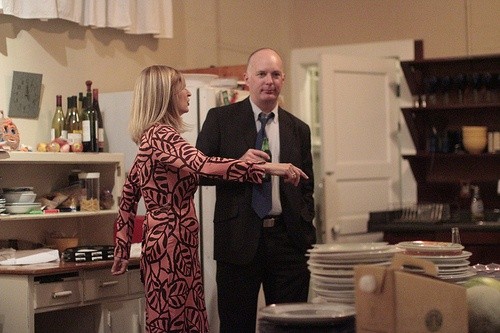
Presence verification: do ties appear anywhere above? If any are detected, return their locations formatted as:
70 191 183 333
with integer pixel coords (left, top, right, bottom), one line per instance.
251 112 275 219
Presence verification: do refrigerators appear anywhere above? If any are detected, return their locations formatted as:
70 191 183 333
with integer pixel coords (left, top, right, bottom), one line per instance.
100 87 249 332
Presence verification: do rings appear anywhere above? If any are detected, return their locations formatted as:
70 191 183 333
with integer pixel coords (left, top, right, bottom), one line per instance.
292 173 296 176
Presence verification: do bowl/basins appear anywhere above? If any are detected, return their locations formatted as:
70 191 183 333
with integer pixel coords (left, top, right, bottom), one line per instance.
46 237 81 252
462 124 488 155
3 187 38 203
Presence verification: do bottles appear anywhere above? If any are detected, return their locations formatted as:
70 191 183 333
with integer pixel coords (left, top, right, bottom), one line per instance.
470 185 484 220
450 226 461 246
259 136 274 183
51 79 106 153
77 171 101 212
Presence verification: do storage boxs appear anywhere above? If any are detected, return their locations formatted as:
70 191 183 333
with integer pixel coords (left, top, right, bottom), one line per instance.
353 256 467 333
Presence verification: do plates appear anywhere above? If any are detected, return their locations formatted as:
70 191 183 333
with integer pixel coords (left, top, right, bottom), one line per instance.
0 198 42 214
257 237 475 324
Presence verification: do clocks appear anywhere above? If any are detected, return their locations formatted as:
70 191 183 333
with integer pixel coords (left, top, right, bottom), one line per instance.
7 70 43 119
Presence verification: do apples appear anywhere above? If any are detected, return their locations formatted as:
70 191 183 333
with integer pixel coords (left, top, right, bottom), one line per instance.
37 141 84 152
460 275 500 333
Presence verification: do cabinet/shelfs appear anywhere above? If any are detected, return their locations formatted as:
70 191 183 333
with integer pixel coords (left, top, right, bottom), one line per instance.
0 258 146 333
0 151 126 219
378 52 500 267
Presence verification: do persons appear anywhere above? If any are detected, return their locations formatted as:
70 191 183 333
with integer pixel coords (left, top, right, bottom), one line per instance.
111 66 270 333
196 48 314 333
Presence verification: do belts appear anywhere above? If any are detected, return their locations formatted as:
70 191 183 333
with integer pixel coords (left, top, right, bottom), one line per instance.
262 215 283 228
